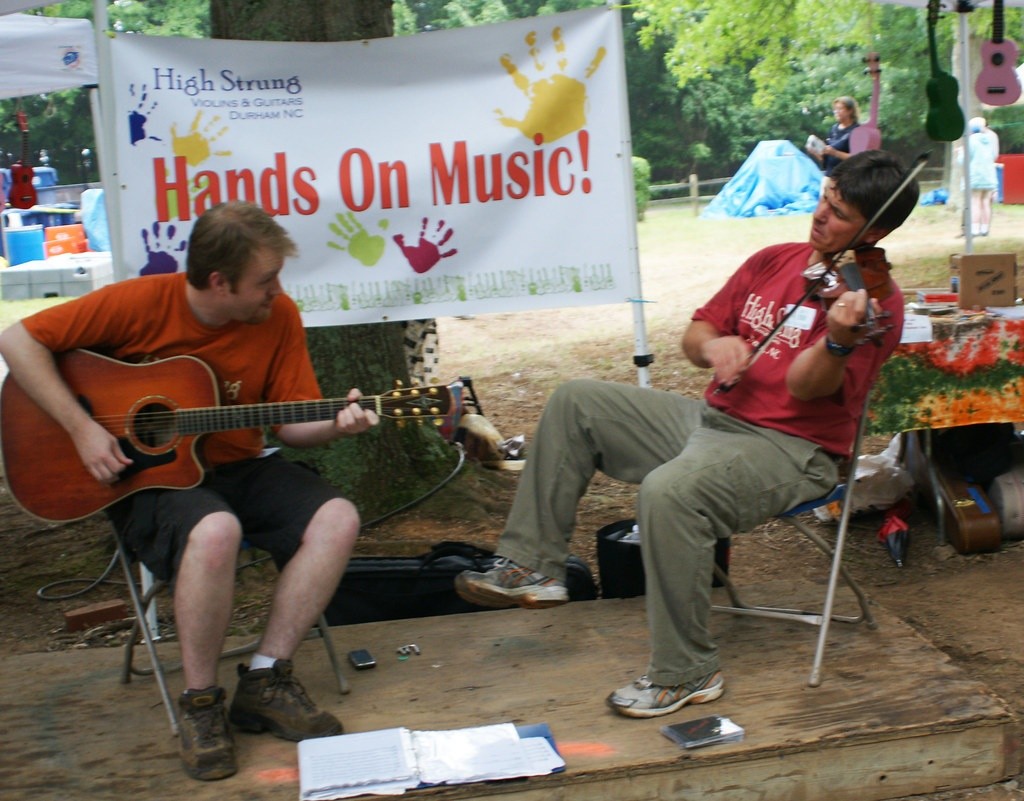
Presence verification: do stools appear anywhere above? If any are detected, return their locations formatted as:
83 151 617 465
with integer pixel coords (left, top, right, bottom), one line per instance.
109 520 351 737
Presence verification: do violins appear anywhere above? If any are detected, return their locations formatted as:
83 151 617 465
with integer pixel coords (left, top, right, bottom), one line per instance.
800 248 895 348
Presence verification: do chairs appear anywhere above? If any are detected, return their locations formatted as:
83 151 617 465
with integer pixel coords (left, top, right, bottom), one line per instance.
712 387 874 688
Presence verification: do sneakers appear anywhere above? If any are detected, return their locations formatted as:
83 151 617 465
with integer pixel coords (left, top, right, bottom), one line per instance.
454 556 571 610
228 658 342 742
607 670 724 718
179 685 236 779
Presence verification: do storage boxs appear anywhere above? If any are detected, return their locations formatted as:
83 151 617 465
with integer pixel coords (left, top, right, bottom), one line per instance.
959 252 1016 310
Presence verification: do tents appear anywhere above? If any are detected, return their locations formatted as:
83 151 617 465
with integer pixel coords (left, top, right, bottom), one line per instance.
702 140 822 218
0 0 126 281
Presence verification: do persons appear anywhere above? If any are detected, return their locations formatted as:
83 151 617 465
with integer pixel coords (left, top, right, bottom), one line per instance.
956 117 999 239
808 99 861 197
454 148 919 718
0 202 382 780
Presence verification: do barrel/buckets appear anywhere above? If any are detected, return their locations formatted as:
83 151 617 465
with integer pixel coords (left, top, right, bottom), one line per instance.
3 224 47 266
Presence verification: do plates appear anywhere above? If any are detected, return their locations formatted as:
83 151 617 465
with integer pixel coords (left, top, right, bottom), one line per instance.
912 305 960 315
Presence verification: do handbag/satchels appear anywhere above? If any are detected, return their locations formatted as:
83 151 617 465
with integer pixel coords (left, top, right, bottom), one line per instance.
594 517 731 598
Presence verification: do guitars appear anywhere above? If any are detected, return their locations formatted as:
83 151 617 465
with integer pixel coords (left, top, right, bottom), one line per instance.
0 347 453 523
923 0 966 142
976 0 1021 106
849 51 883 158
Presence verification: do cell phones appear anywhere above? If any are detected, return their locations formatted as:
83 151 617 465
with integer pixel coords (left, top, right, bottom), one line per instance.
348 649 377 670
